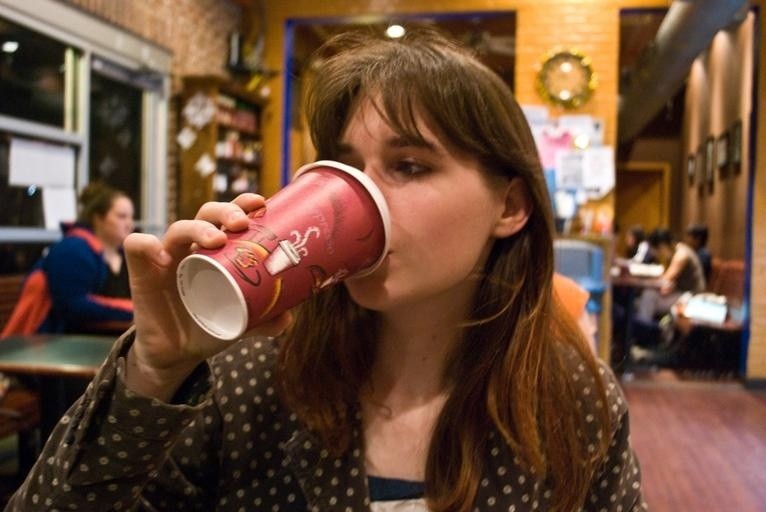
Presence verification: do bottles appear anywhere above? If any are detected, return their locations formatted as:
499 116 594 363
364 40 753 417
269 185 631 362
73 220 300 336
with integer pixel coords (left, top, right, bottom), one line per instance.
215 95 258 133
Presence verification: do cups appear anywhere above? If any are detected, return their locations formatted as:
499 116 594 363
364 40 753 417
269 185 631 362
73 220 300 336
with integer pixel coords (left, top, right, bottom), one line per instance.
176 158 391 348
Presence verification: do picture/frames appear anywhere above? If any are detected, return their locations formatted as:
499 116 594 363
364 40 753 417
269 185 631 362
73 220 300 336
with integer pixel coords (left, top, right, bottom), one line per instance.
682 119 741 204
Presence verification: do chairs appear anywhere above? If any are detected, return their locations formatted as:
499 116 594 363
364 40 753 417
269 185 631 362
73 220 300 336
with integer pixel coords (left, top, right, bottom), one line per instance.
0 388 46 476
662 259 748 378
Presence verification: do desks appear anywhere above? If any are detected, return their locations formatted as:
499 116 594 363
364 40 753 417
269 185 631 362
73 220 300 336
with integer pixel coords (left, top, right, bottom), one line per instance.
611 268 669 367
1 331 121 461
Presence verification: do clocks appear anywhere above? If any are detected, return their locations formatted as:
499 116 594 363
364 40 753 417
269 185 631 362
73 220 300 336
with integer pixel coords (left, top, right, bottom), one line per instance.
535 46 597 109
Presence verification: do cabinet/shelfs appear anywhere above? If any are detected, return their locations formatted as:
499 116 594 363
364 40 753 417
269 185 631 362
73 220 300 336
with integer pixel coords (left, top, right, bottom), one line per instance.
180 75 264 217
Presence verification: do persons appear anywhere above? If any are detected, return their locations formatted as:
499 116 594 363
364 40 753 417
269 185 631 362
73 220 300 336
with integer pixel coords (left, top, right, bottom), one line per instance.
4 22 650 510
607 208 714 369
2 179 138 332
